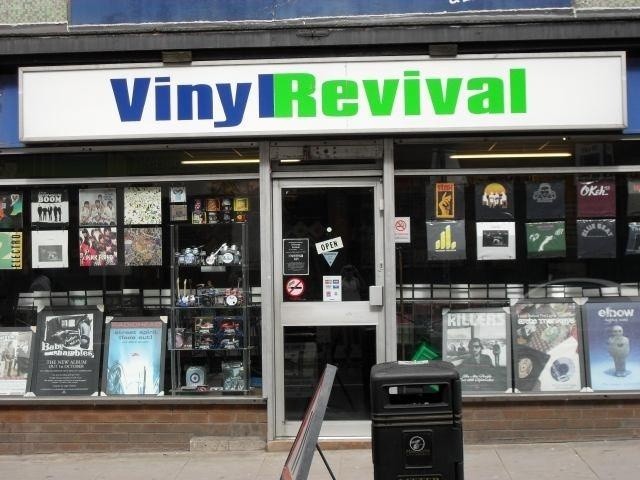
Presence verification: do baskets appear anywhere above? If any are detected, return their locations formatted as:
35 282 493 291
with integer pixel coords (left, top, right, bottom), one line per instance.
411 342 441 361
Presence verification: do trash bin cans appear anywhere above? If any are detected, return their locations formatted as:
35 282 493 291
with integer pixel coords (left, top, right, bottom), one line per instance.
370 361 464 480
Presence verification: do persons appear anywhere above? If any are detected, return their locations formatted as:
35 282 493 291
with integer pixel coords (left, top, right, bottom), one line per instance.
493 341 501 365
4 342 15 379
439 192 451 215
78 316 91 349
462 338 492 365
38 195 117 266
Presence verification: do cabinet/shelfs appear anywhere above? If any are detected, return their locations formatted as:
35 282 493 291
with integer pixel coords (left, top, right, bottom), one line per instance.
170 222 255 397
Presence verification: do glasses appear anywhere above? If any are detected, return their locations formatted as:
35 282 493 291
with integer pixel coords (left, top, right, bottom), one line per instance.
470 345 480 350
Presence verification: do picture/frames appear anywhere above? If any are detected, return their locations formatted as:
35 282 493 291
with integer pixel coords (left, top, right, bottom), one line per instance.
0 304 169 398
442 298 639 394
